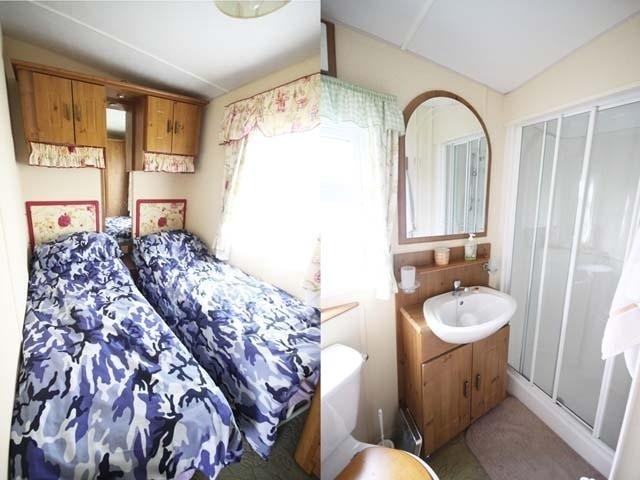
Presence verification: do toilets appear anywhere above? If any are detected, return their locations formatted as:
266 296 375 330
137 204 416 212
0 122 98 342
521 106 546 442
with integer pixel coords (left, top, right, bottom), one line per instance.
320 343 442 480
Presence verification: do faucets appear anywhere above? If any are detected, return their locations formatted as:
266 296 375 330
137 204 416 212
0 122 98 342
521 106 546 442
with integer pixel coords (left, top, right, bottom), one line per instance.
452 280 471 295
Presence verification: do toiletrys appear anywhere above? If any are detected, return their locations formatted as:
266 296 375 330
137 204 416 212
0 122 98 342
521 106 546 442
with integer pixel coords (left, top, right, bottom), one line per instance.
465 233 479 261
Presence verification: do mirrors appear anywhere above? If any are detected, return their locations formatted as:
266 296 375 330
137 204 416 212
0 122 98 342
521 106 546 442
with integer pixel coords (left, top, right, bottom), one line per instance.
399 89 490 241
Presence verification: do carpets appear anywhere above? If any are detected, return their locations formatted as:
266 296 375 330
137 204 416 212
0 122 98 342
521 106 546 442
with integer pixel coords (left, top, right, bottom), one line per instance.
464 395 608 480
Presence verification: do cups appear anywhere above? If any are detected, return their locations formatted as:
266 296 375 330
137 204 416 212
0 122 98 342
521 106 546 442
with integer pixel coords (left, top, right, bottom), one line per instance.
435 248 450 265
401 264 416 286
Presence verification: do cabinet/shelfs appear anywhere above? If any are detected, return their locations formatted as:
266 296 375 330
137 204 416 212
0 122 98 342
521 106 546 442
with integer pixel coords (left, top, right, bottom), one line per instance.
145 96 201 157
420 327 510 453
31 73 106 147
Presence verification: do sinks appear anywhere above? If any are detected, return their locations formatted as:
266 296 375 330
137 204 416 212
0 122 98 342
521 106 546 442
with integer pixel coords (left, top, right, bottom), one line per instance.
422 284 516 343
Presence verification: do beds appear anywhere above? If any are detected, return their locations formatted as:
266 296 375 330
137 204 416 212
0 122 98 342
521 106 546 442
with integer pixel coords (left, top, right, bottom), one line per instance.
10 198 322 480
